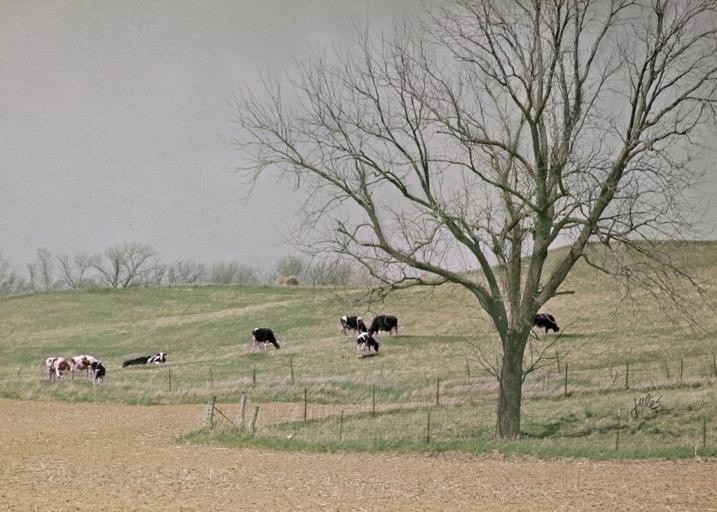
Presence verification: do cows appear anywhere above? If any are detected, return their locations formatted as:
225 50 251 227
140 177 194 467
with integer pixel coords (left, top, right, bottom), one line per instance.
89 360 107 386
356 331 380 360
146 352 167 365
368 314 399 336
529 312 561 335
122 355 151 367
251 326 281 353
339 314 368 336
45 356 74 384
255 339 265 342
70 354 98 378
